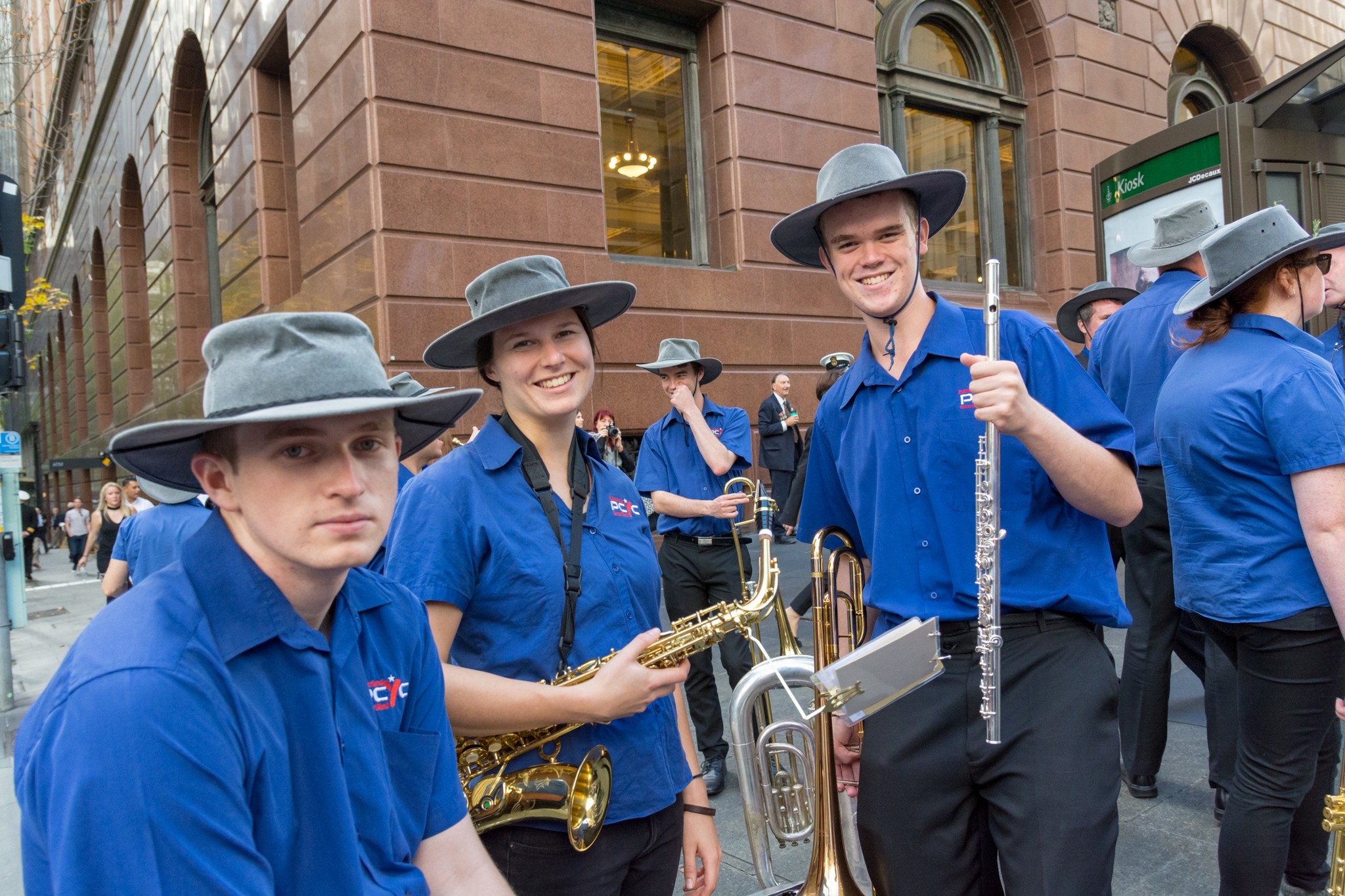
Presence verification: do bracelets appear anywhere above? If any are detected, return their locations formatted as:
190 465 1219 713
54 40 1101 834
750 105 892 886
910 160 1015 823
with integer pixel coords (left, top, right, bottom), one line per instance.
81 554 88 557
683 802 716 817
692 774 703 779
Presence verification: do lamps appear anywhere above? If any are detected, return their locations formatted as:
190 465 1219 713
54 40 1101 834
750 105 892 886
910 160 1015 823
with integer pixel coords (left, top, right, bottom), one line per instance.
605 44 660 182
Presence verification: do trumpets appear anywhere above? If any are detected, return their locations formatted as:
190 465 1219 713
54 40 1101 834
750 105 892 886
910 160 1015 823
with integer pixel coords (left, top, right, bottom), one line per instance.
785 526 866 896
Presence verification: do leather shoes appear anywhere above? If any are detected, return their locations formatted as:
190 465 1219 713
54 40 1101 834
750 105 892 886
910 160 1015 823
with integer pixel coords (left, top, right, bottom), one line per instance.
774 529 797 544
1118 758 1159 798
771 755 790 786
700 758 728 794
1213 787 1231 822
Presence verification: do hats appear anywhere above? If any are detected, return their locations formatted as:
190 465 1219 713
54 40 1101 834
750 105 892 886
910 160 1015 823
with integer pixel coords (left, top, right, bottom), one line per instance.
819 352 854 372
636 337 723 386
18 490 31 501
1172 204 1345 316
1313 222 1345 251
1056 281 1141 344
1127 198 1227 272
422 254 637 374
107 310 485 506
767 143 967 268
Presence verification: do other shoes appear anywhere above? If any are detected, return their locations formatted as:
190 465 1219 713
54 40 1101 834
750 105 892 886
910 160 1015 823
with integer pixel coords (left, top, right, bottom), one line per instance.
1278 873 1330 896
32 563 40 568
25 577 32 583
45 546 60 553
72 565 84 574
775 614 802 648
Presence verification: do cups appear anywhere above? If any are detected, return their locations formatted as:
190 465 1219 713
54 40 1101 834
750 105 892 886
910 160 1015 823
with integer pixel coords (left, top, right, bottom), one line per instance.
790 409 798 417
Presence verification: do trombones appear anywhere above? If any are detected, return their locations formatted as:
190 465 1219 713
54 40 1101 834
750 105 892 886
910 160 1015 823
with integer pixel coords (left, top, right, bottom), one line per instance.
721 479 802 788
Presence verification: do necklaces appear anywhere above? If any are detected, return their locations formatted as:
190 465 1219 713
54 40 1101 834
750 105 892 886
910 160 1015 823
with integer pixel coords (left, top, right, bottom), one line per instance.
107 506 121 510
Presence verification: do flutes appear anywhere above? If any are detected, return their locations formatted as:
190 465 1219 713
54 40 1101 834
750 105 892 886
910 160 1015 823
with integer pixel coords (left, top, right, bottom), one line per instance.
978 256 1006 742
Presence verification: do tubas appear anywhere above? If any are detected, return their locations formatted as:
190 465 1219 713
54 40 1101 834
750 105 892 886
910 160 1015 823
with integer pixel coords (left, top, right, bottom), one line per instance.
728 654 871 896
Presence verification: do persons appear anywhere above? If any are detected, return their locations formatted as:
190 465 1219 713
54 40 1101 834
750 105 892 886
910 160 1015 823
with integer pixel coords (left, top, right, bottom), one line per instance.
384 256 721 896
758 372 804 546
1153 202 1345 896
574 410 584 428
32 526 41 570
363 370 482 575
34 505 49 554
586 410 636 475
1309 221 1345 385
75 483 134 607
14 313 524 895
64 501 75 562
63 497 94 574
1054 279 1139 687
1086 192 1239 822
18 488 38 581
101 473 216 598
122 479 154 512
778 369 846 648
772 145 1143 895
48 507 63 550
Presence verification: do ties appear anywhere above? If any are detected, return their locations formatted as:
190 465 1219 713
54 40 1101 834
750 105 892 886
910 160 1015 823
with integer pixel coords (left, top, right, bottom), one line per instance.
784 402 799 443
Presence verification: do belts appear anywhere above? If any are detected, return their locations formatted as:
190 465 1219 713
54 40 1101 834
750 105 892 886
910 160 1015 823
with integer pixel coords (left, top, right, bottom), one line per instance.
937 607 1096 637
664 532 752 545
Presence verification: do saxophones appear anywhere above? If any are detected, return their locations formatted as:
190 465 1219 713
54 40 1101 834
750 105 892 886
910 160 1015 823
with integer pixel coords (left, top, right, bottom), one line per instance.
447 477 781 851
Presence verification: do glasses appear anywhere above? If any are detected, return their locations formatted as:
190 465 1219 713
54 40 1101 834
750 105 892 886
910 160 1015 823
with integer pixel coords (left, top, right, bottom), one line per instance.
1284 253 1332 275
